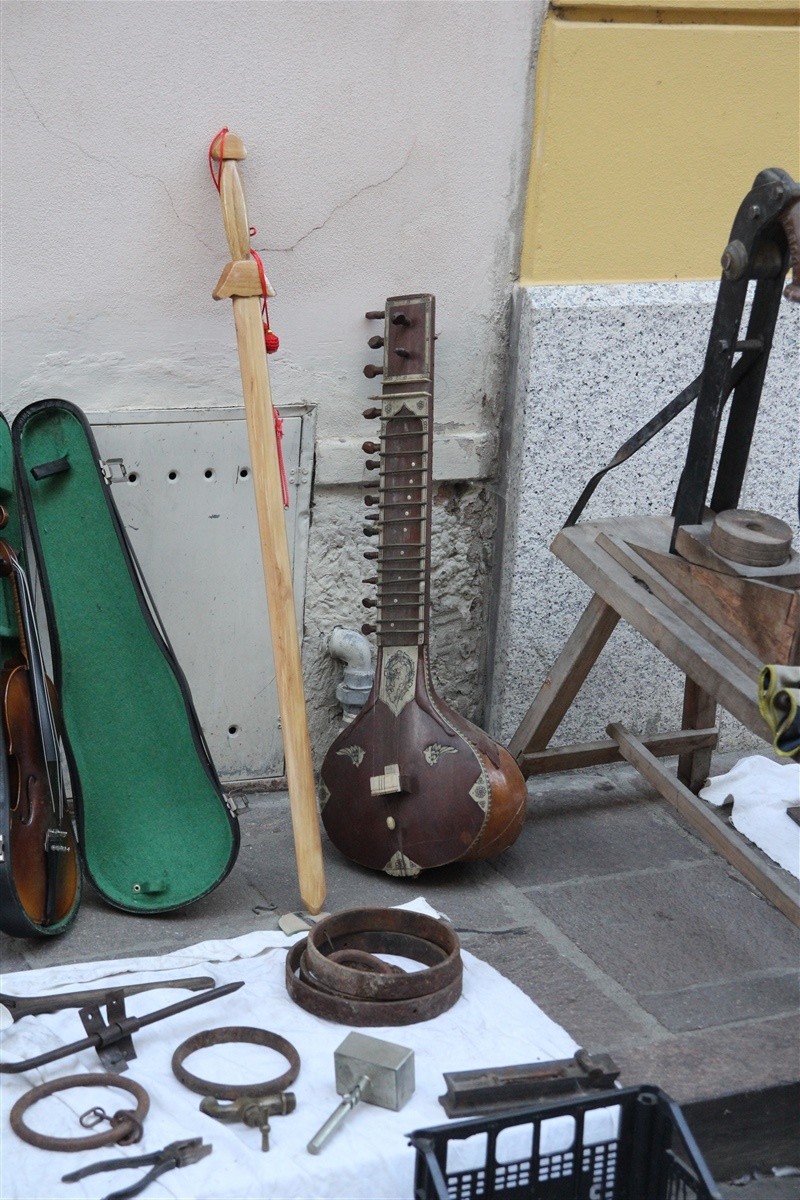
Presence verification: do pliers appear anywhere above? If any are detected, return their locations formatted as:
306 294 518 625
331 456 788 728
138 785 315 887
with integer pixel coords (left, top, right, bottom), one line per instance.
61 1137 213 1200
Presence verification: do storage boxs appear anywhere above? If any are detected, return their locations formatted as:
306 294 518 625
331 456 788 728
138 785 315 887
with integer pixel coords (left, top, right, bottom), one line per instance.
405 1082 723 1200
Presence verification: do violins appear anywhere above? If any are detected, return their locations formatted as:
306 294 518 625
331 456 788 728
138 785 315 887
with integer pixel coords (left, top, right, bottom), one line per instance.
0 504 77 927
